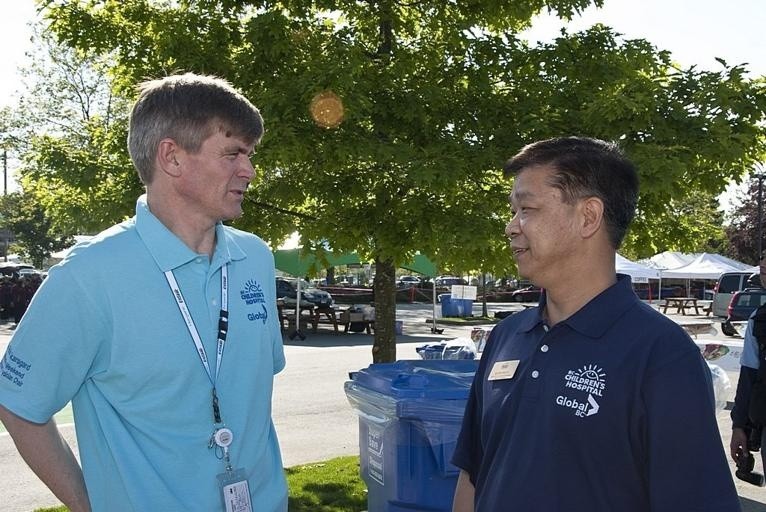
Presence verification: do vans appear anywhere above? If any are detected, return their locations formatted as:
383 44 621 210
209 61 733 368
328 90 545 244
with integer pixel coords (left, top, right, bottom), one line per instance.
713 271 755 318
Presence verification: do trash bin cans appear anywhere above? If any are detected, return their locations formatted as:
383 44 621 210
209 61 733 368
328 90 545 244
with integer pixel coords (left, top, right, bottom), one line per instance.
343 345 480 512
438 293 473 317
395 321 403 335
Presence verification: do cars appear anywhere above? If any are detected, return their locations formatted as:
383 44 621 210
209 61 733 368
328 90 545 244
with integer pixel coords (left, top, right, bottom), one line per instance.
511 284 541 302
726 288 766 334
276 276 332 308
397 274 467 290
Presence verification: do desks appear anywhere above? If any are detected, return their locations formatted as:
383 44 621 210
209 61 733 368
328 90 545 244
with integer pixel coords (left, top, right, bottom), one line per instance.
277 303 375 335
661 297 714 316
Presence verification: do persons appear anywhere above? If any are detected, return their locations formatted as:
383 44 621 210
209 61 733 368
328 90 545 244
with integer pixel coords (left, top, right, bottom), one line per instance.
0 72 291 512
449 138 740 512
730 250 765 486
